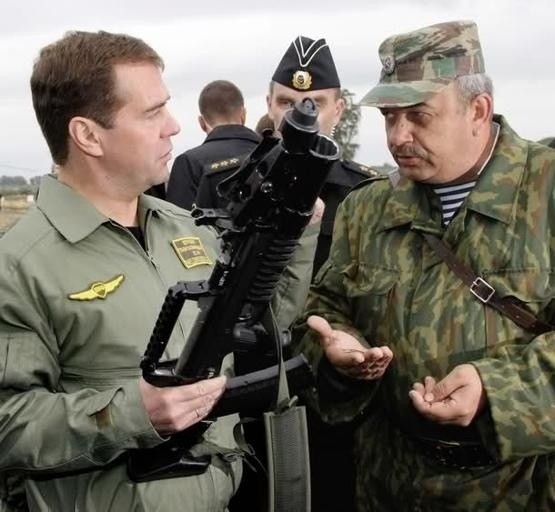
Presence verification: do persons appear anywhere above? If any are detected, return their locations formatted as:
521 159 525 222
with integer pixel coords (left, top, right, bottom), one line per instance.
0 30 327 512
165 80 260 210
254 114 275 134
192 35 380 512
292 19 555 512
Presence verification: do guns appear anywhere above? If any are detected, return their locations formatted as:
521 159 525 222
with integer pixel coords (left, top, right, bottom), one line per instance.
126 96 341 483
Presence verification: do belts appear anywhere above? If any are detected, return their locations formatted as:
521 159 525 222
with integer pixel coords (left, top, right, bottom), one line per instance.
361 413 496 468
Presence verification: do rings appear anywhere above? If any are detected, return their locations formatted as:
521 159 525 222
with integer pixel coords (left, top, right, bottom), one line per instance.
195 410 201 417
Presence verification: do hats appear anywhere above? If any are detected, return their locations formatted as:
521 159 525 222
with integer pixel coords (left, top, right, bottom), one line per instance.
357 21 486 109
272 35 340 92
